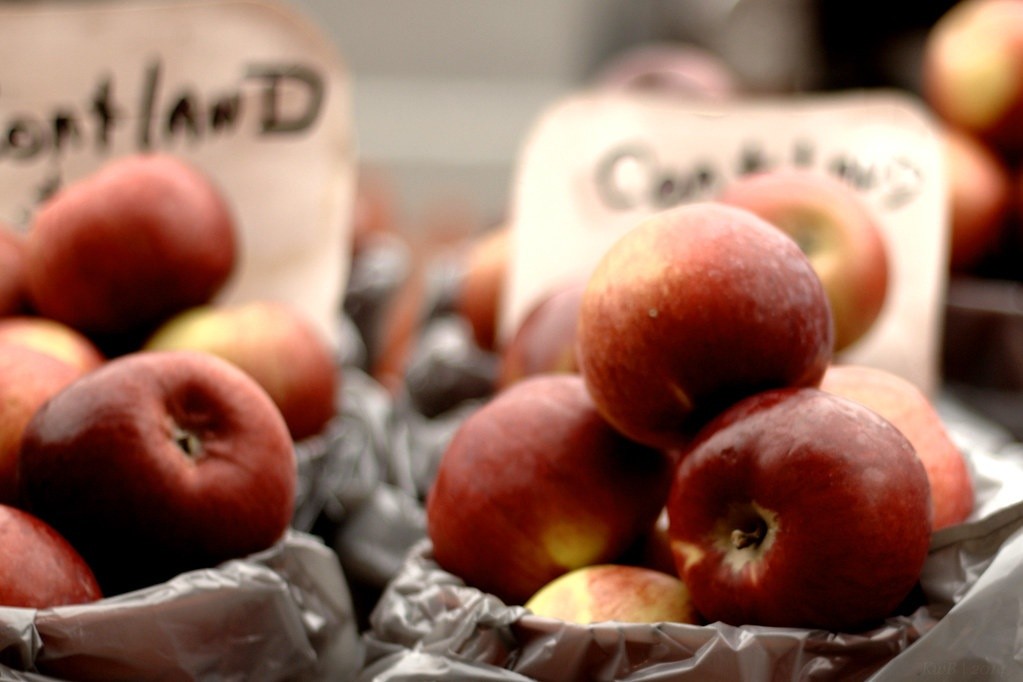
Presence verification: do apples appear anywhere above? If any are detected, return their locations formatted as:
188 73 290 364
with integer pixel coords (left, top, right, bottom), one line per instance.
0 0 1023 630
